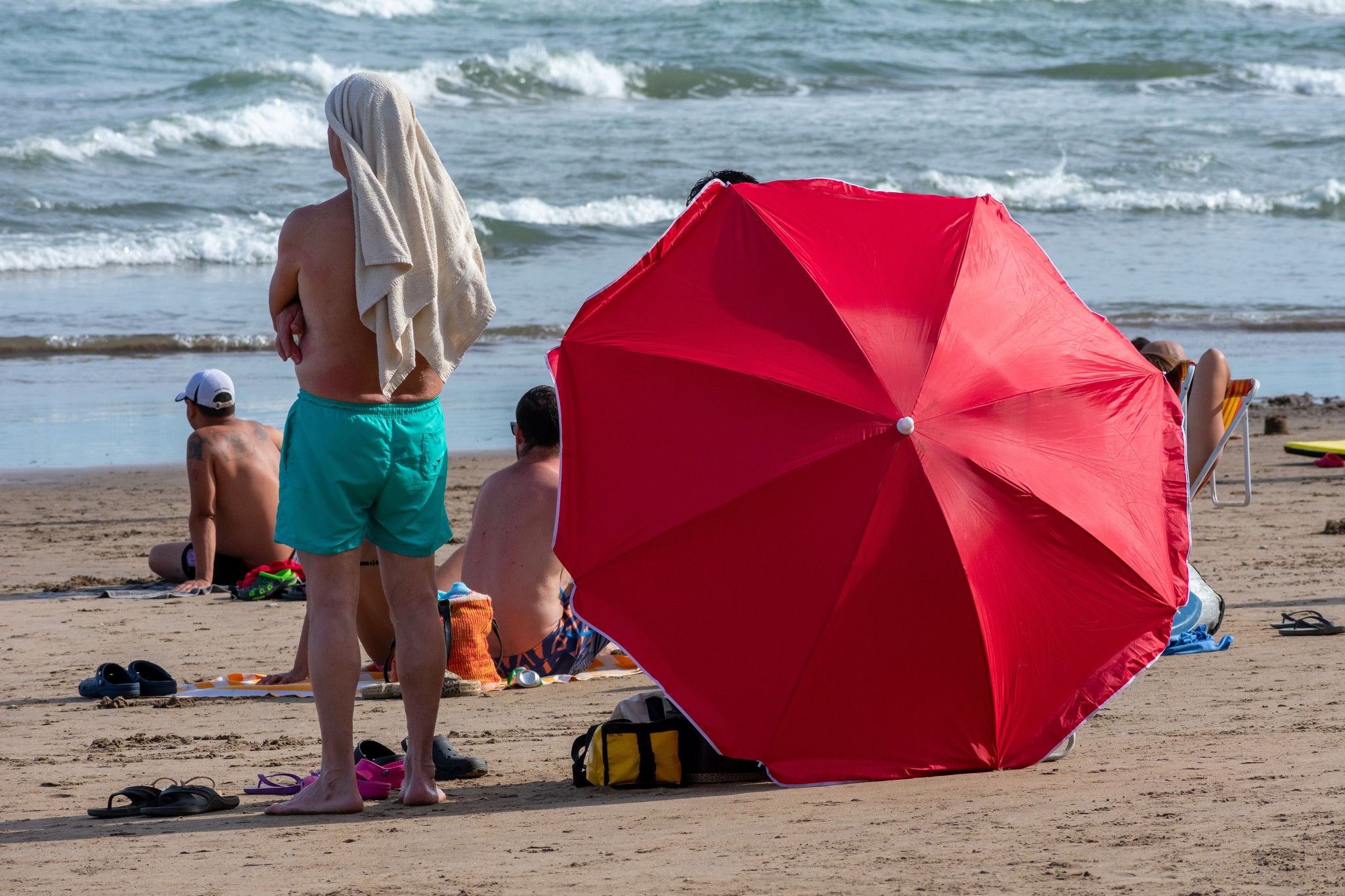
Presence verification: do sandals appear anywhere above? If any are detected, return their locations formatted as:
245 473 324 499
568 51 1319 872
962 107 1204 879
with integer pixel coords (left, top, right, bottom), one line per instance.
238 568 307 601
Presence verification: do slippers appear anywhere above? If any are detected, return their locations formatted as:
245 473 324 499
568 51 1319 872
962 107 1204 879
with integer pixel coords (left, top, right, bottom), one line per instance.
1271 609 1345 635
300 775 391 800
1314 452 1345 467
401 734 489 779
355 759 405 789
243 769 321 794
127 660 178 694
140 776 240 816
355 739 407 766
88 777 177 817
360 668 481 697
79 662 140 697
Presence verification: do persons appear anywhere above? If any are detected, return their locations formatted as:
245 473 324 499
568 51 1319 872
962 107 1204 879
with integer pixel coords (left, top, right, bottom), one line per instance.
255 537 467 686
147 368 295 594
267 69 499 814
685 170 758 208
462 385 612 680
1130 337 1231 488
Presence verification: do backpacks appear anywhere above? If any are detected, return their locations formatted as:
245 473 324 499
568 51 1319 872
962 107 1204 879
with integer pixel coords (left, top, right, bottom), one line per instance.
570 696 689 789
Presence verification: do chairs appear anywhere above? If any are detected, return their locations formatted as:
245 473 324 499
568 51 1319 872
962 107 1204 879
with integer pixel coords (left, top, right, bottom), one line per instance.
1187 377 1260 507
1171 356 1196 438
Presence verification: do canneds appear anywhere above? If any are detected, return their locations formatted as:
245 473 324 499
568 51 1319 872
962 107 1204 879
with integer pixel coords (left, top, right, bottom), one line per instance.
508 666 543 688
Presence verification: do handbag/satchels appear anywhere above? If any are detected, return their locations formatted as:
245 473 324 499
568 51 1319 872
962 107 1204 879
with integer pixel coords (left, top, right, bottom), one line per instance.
381 590 504 690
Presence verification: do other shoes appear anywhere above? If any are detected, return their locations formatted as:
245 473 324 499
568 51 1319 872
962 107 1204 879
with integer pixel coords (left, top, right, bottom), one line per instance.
686 753 766 783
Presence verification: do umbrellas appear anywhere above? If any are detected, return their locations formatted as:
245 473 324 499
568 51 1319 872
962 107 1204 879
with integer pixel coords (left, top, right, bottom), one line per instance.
548 179 1192 785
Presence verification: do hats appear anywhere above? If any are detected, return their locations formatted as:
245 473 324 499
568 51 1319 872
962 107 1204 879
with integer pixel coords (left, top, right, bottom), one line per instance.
175 368 235 410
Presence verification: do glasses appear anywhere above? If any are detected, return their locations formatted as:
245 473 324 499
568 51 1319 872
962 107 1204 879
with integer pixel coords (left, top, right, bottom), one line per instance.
510 421 518 436
